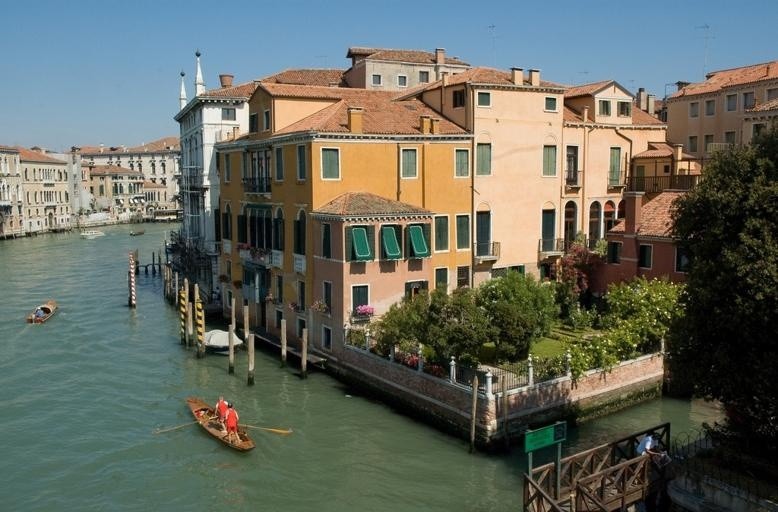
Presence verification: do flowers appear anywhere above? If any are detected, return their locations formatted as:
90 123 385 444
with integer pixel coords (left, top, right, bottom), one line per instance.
310 301 328 313
356 305 375 316
287 303 297 313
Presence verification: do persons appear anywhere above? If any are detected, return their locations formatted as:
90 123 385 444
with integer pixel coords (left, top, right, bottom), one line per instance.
35 306 44 318
224 402 240 444
214 395 228 430
636 431 660 484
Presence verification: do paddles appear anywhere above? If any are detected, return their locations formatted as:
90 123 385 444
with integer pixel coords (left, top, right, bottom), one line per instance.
236 423 290 435
154 415 219 434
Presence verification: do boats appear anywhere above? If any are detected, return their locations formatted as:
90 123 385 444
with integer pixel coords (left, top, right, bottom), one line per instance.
203 328 244 355
187 396 255 451
81 229 105 239
26 300 57 324
